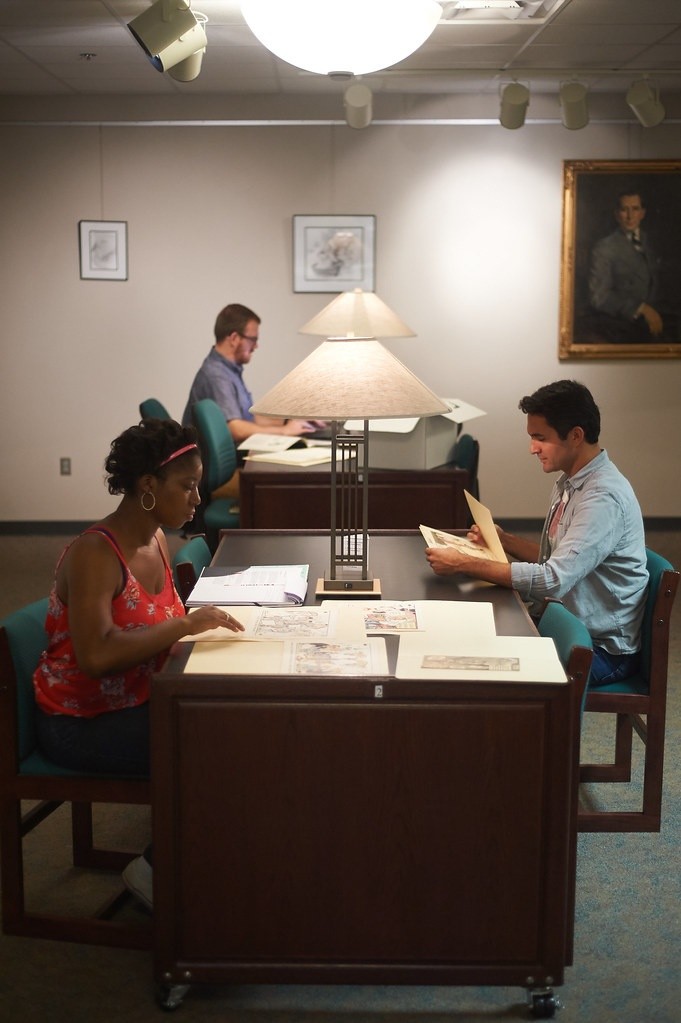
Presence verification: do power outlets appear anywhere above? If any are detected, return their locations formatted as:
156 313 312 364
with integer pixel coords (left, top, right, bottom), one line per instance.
60 458 70 475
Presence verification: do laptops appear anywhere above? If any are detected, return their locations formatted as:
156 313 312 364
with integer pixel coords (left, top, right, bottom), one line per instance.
301 420 345 440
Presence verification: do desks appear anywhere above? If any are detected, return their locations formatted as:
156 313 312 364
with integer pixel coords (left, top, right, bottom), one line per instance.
241 421 470 530
150 531 575 1017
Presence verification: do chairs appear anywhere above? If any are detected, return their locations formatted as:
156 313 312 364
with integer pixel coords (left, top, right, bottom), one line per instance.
0 597 151 951
456 434 480 528
140 399 170 425
536 597 594 757
193 399 243 531
577 545 681 832
173 534 212 615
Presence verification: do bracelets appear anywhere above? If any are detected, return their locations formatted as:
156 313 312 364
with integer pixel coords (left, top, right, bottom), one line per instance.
284 418 289 425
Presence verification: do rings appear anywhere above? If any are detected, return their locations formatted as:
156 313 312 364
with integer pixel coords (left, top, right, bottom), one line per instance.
224 613 230 621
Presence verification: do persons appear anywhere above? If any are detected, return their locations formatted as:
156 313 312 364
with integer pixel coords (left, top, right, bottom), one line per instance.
32 417 245 913
423 378 650 687
180 303 328 447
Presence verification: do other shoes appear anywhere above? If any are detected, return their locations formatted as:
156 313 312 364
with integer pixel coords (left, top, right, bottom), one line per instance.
123 856 154 911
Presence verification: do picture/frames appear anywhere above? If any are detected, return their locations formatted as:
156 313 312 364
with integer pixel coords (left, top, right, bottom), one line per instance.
558 159 681 360
292 214 376 293
79 220 128 281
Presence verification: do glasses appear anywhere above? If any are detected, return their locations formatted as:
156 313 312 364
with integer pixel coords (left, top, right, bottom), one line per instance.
238 333 258 344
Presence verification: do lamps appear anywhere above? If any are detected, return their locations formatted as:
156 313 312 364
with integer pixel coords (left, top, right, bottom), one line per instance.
249 337 449 598
498 80 531 129
295 288 418 424
343 75 372 129
235 0 442 81
625 73 666 128
127 0 208 82
559 70 590 129
